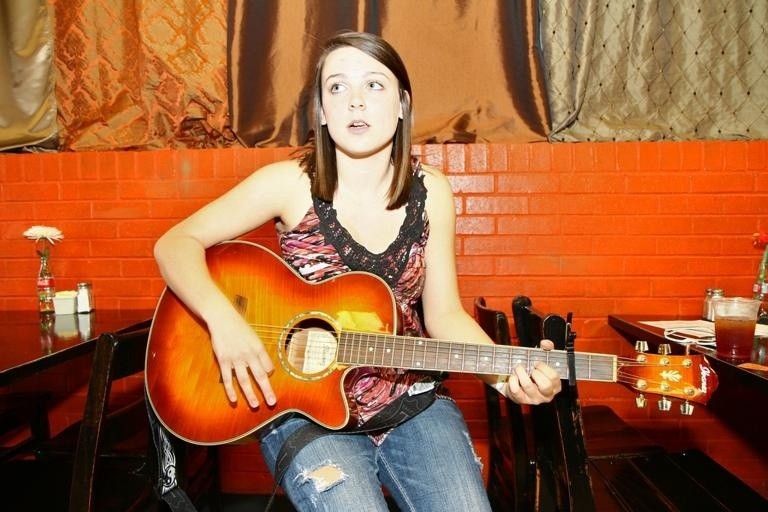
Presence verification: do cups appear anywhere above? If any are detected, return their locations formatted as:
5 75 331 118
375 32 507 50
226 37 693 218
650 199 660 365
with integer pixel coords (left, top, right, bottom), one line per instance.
710 297 764 363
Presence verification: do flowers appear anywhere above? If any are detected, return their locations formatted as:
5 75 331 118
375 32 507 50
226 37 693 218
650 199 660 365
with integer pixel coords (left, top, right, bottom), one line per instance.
21 225 66 255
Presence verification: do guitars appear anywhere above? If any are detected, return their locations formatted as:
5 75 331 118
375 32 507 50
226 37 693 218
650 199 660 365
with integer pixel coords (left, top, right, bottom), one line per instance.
145 239 720 446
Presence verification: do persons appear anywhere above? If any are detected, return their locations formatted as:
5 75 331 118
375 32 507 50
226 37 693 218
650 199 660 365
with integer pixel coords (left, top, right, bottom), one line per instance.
152 29 565 512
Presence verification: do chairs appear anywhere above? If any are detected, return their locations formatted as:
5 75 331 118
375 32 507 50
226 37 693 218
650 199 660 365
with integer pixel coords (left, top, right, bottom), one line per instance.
513 297 768 512
64 327 161 512
474 296 656 512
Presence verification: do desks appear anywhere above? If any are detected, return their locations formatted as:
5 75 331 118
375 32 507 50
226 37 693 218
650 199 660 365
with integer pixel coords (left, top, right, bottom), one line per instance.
608 315 768 389
0 309 158 431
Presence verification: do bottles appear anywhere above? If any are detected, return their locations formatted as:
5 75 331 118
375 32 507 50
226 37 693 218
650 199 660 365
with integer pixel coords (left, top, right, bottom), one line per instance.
751 262 768 325
36 256 57 313
77 314 94 341
76 282 93 313
701 287 724 322
38 314 56 355
